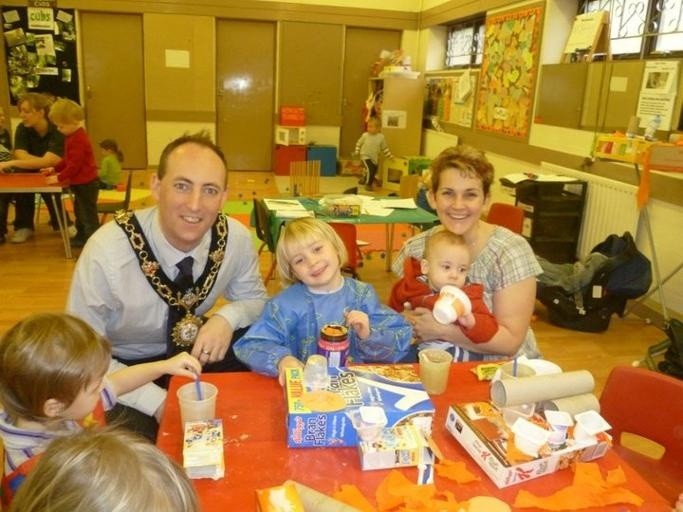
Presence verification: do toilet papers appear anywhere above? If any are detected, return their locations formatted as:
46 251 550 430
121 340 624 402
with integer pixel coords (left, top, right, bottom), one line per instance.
489 368 600 407
546 392 602 417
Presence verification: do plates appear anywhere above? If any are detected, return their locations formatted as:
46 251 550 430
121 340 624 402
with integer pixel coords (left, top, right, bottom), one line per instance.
491 359 562 383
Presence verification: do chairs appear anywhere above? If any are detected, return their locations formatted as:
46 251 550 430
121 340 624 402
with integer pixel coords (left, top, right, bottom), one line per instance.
329 223 360 280
96 174 133 224
487 204 524 233
600 366 683 507
253 198 278 287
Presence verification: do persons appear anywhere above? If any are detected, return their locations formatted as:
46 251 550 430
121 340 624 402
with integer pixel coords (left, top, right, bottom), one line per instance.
390 145 543 362
355 118 396 191
0 107 13 241
0 94 77 243
10 426 197 511
40 99 101 248
0 314 203 487
95 139 124 189
390 230 498 363
64 132 268 443
232 218 413 387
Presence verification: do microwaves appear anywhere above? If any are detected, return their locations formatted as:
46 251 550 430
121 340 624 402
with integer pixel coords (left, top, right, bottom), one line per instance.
274 124 307 147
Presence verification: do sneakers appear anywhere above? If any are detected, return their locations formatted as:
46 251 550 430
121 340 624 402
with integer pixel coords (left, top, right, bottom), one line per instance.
11 228 32 243
67 226 78 237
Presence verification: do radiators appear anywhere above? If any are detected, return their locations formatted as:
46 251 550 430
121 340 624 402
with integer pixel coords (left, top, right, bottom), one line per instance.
540 161 642 260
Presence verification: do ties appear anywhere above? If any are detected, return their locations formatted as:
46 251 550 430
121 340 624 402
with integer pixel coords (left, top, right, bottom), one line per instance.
167 255 197 360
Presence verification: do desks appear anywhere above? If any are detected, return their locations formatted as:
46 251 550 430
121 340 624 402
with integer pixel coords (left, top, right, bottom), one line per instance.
155 360 676 512
0 173 72 259
250 197 439 271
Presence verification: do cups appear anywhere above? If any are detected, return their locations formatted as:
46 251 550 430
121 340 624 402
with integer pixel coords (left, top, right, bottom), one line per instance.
418 348 453 395
573 410 612 444
359 406 388 441
544 410 575 442
176 381 218 433
500 363 536 379
511 417 551 457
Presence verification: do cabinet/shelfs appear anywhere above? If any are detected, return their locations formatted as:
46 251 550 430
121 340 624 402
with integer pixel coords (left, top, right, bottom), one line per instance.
515 181 587 264
368 78 424 182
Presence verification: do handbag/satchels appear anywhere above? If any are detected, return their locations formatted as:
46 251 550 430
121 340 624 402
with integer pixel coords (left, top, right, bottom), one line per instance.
536 232 654 331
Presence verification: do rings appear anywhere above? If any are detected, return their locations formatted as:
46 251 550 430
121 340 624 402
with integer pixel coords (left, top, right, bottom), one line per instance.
201 350 214 355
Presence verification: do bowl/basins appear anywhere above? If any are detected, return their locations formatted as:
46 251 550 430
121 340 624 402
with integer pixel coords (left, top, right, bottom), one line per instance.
432 285 472 325
303 354 328 383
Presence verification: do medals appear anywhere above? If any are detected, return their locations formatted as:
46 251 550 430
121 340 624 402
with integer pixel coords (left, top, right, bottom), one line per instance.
113 210 227 349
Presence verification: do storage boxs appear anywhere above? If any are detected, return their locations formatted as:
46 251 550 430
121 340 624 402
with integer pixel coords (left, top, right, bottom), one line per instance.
308 146 336 177
280 106 305 126
273 145 307 176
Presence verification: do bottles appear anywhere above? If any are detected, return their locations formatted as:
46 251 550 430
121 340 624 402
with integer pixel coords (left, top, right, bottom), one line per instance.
625 117 641 138
644 118 661 142
317 324 351 367
